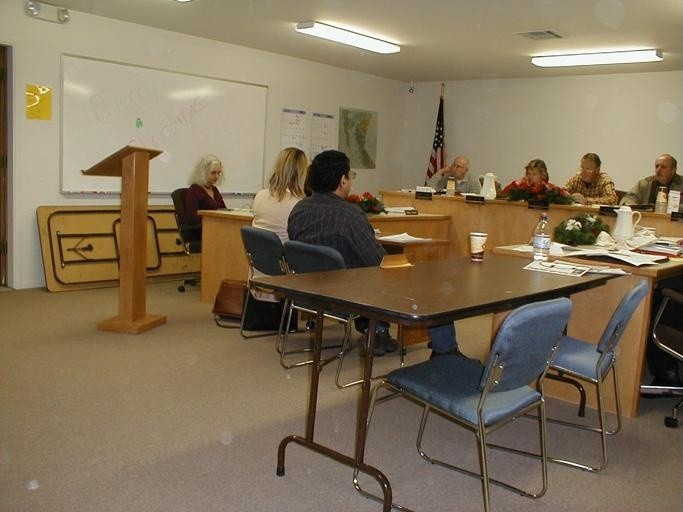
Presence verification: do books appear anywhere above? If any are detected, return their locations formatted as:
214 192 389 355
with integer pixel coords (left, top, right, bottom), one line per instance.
378 254 415 270
584 235 683 267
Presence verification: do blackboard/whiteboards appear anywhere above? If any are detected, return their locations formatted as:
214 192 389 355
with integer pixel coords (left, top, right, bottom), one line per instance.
59 52 269 197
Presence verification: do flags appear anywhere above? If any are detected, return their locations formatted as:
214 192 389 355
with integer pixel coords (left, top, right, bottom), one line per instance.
424 96 445 185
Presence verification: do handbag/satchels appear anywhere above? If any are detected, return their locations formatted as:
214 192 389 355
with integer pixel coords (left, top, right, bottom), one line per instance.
212 278 247 319
244 292 297 330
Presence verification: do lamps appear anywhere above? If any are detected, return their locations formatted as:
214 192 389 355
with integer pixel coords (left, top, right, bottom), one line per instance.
295 21 400 58
531 49 666 69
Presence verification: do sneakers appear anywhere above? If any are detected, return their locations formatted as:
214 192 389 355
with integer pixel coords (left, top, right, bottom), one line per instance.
373 336 398 357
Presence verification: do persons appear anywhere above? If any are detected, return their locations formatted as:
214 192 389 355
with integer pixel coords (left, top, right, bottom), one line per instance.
287 150 481 363
183 154 228 250
426 155 481 194
620 153 682 206
501 159 566 197
561 153 618 206
251 147 310 294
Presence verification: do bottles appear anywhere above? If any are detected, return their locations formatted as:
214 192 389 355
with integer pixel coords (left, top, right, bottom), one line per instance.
533 213 551 262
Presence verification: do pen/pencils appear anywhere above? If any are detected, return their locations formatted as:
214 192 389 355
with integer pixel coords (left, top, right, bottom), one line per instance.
562 189 572 197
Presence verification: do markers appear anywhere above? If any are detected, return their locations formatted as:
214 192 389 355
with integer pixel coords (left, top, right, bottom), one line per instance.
81 191 96 194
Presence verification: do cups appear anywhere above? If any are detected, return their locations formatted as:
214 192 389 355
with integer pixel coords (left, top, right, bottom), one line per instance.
470 232 488 262
446 177 456 198
655 186 668 214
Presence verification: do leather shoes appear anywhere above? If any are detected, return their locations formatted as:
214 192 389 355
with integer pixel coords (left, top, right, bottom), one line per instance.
640 374 681 398
